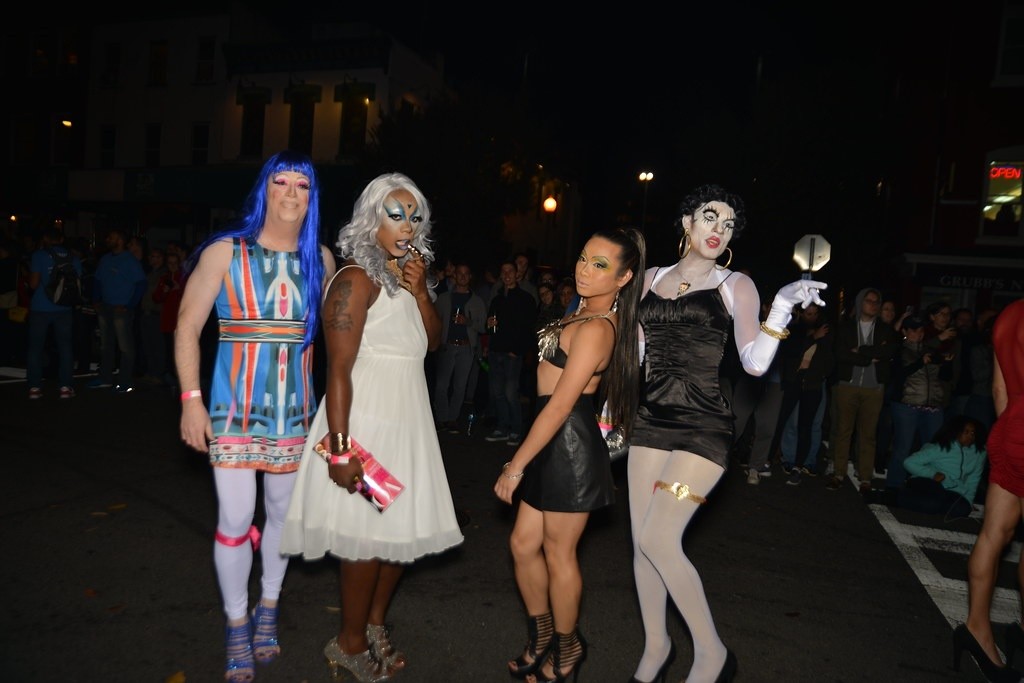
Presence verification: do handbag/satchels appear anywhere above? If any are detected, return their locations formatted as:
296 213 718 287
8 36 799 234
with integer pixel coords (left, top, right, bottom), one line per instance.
0 290 17 309
8 306 29 324
82 281 98 315
606 423 629 462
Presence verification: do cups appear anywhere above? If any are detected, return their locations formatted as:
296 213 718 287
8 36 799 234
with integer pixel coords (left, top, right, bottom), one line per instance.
489 312 500 333
453 307 462 323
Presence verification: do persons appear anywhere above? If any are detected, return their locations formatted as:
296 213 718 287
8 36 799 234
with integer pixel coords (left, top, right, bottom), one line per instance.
26 228 82 398
627 184 828 683
433 253 578 446
494 226 645 683
86 227 148 391
0 225 201 372
953 300 1024 683
722 270 999 522
175 154 335 683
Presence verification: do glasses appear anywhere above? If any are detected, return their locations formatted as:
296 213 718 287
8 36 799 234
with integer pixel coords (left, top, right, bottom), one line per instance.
540 290 551 297
542 279 552 284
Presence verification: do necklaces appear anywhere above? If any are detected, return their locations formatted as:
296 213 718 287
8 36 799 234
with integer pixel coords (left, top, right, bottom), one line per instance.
675 266 713 297
536 310 616 364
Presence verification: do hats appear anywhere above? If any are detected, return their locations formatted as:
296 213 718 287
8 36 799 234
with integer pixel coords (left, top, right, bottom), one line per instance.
899 314 927 330
857 287 881 305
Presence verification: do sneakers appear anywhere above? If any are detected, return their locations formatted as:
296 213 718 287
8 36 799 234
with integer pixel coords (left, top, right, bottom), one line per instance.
485 430 509 441
506 432 522 446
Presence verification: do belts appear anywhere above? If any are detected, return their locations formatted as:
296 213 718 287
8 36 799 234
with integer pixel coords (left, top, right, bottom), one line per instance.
447 339 466 345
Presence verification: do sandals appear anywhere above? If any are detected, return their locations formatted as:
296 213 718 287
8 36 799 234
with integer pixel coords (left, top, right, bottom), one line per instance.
250 601 280 663
224 621 254 683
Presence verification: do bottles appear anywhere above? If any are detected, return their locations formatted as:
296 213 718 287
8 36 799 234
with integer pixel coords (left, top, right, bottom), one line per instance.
479 359 490 372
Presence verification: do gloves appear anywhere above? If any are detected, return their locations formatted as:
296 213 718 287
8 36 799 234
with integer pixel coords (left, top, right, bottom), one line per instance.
740 279 828 376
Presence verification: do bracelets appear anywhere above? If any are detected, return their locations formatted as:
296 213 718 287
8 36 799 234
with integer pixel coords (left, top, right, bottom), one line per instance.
180 390 202 400
760 321 790 340
503 462 524 479
329 432 351 456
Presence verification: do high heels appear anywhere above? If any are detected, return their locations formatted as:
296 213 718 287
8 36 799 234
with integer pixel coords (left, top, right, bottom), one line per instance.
524 625 588 683
715 647 737 683
626 638 676 683
324 636 392 683
952 623 1021 683
508 609 556 680
364 624 408 672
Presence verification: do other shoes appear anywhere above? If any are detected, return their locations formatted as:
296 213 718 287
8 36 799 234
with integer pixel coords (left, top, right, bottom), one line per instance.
733 458 901 496
17 363 147 394
450 428 460 434
967 504 985 519
1002 541 1022 563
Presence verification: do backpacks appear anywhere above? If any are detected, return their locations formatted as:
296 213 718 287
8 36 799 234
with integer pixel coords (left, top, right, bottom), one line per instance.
44 269 82 307
